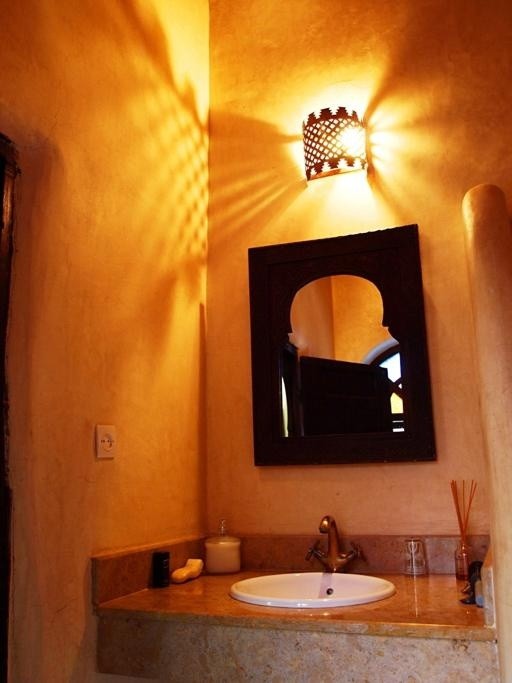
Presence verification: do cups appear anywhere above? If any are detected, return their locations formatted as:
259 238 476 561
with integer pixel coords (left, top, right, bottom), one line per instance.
403 539 429 576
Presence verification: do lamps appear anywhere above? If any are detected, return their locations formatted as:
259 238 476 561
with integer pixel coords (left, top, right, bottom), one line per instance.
302 106 369 181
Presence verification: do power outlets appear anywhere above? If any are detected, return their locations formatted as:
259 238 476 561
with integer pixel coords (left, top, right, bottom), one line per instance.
95 425 117 459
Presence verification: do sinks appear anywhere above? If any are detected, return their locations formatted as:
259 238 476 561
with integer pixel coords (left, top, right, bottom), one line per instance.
231 572 397 608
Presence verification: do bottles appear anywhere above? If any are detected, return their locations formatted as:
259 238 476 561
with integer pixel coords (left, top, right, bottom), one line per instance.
454 540 473 576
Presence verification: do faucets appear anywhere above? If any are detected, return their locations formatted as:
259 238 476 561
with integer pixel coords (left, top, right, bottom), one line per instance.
320 515 342 570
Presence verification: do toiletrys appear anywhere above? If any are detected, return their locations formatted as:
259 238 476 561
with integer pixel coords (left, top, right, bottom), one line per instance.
204 517 242 574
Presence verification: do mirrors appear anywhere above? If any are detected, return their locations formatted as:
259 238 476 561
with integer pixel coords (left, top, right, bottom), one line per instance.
249 223 438 465
